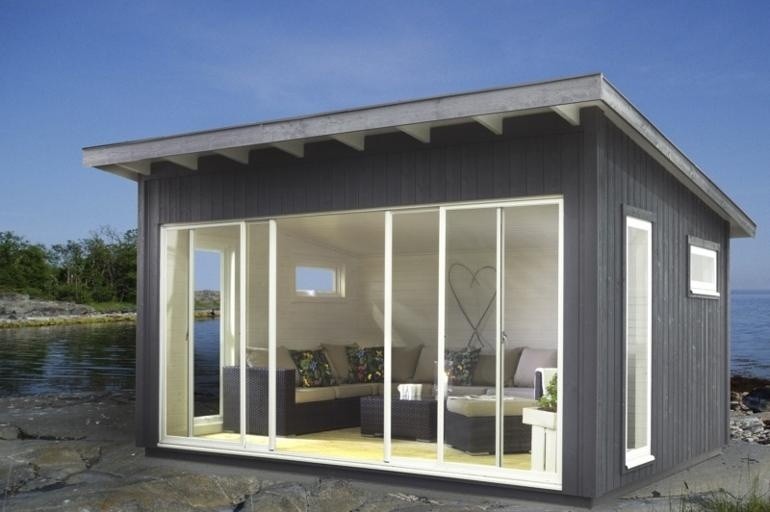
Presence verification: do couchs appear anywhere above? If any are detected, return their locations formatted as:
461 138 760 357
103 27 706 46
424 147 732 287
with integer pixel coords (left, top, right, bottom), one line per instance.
223 365 535 437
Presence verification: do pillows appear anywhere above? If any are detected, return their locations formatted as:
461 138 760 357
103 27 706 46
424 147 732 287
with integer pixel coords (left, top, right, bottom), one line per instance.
288 347 340 386
345 346 384 384
444 346 481 386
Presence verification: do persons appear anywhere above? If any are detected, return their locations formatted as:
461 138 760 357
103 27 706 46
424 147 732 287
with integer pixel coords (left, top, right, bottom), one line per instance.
207 308 215 316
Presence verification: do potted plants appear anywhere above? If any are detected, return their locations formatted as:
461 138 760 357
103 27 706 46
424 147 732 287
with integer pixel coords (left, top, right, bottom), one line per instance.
523 372 557 473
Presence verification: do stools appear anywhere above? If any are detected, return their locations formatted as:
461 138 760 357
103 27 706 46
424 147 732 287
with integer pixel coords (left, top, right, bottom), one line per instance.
446 397 535 453
